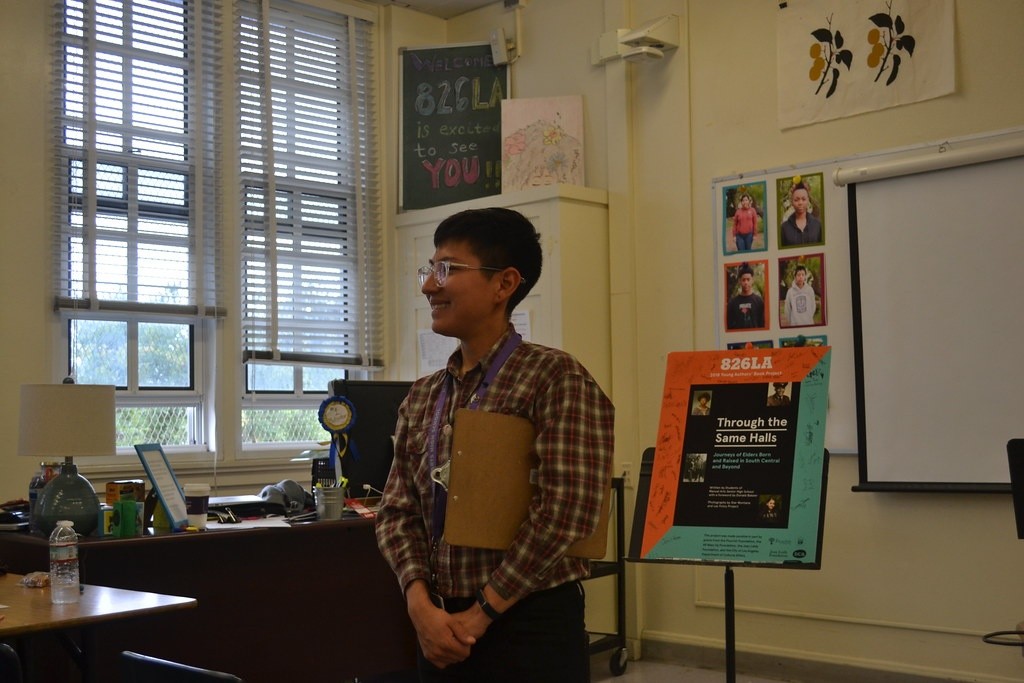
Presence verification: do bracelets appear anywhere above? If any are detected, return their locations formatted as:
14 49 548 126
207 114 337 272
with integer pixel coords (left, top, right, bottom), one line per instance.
476 588 503 621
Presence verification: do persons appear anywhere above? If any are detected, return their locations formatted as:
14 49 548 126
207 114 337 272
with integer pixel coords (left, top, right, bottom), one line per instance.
727 264 765 328
688 455 703 482
732 195 758 251
762 496 777 518
374 207 615 683
693 392 710 415
786 266 816 326
768 382 789 405
781 181 822 245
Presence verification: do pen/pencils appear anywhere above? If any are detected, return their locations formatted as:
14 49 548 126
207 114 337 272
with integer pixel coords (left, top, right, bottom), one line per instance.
315 476 348 488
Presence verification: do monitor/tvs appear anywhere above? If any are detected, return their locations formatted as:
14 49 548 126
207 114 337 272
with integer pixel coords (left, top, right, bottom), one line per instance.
328 379 415 497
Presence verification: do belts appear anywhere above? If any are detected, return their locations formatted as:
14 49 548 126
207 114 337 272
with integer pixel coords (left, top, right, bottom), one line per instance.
431 582 582 613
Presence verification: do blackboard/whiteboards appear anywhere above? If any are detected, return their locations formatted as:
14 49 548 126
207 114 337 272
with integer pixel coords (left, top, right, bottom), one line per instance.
398 40 511 214
711 123 1024 455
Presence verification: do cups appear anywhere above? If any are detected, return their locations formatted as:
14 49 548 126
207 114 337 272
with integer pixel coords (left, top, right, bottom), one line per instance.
183 484 210 529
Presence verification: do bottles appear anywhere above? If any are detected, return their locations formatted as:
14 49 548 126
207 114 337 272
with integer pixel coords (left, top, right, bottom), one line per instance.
120 489 137 536
56 521 79 604
28 470 43 533
49 521 63 605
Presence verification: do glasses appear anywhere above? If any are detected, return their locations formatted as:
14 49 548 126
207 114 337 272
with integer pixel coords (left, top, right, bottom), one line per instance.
417 261 525 287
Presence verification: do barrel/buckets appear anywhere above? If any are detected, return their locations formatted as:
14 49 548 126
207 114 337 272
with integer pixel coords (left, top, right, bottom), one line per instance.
312 487 345 520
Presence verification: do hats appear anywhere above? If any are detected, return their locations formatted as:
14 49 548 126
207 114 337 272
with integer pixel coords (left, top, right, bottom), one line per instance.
773 382 788 388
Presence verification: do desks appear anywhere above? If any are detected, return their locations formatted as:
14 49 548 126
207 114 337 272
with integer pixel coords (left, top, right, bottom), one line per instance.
1 495 429 682
